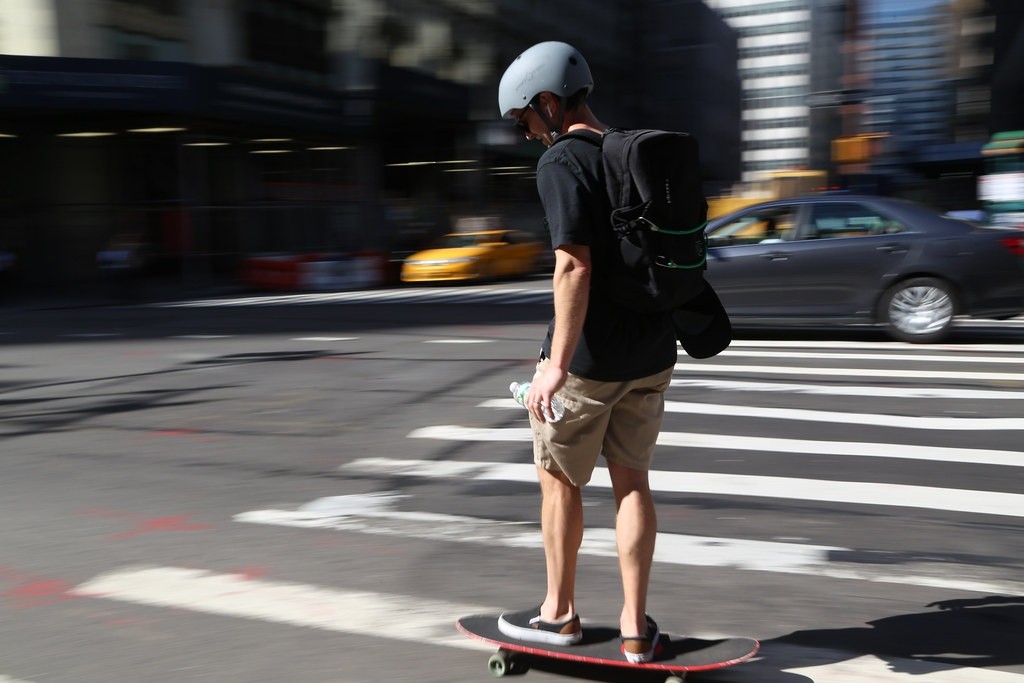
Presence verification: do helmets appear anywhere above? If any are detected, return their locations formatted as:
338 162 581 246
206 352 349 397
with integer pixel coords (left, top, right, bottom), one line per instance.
497 41 594 120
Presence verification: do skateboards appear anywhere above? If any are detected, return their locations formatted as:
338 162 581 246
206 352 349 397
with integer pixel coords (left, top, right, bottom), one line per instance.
455 614 760 682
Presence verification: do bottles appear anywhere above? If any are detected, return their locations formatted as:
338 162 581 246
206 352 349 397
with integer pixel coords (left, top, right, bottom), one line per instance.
510 382 565 423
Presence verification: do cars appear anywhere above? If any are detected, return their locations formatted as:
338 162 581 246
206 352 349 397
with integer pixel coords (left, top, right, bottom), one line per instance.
401 231 547 286
707 194 1024 344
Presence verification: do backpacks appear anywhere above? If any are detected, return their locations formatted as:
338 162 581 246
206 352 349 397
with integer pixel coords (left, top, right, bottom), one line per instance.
548 128 732 359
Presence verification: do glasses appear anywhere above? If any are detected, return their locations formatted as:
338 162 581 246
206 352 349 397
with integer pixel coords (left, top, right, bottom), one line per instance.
512 105 531 137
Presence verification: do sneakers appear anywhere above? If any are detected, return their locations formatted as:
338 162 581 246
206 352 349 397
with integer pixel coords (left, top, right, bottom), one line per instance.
497 602 583 645
620 614 659 662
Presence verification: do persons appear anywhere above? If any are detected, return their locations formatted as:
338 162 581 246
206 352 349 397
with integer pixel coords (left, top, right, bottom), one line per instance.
497 41 679 663
